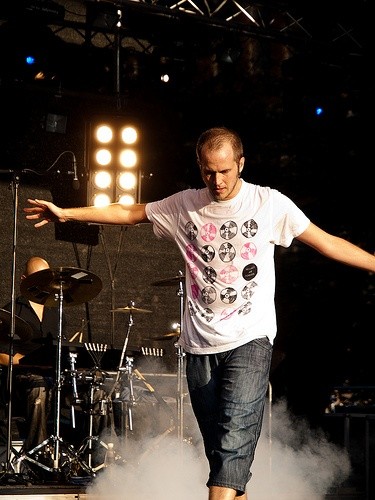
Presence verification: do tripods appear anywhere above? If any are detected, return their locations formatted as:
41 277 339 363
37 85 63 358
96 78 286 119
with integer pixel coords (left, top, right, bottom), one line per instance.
0 184 176 486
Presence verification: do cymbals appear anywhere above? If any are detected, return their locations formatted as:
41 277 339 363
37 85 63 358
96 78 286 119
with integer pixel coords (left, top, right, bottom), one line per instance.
0 308 33 345
20 266 103 308
109 307 153 313
153 329 181 341
150 276 186 287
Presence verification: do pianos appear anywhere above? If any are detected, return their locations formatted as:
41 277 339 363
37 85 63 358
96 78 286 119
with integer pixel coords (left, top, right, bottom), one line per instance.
19 340 181 480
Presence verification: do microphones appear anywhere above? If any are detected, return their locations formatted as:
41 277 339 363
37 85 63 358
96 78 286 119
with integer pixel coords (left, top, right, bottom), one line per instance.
71 155 81 191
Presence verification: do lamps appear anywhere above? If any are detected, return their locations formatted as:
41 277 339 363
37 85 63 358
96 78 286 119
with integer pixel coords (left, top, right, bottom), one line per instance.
80 120 142 228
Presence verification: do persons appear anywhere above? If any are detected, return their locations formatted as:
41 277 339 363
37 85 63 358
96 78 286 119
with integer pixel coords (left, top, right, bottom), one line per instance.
0 256 87 459
24 127 375 500
